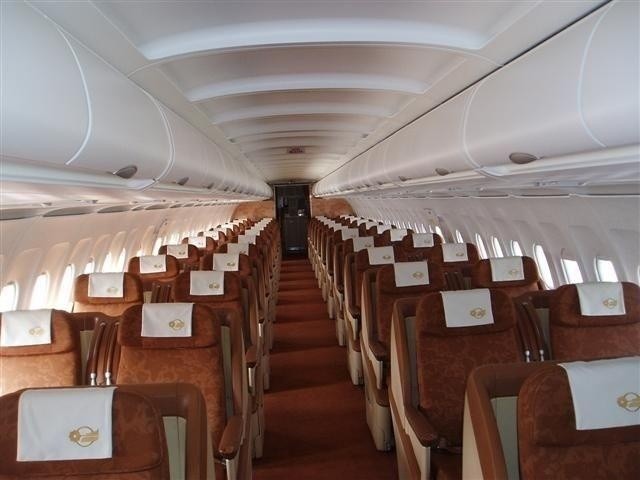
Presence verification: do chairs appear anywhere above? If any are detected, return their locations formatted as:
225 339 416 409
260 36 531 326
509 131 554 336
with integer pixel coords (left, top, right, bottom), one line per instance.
307 213 640 480
0 216 282 480
